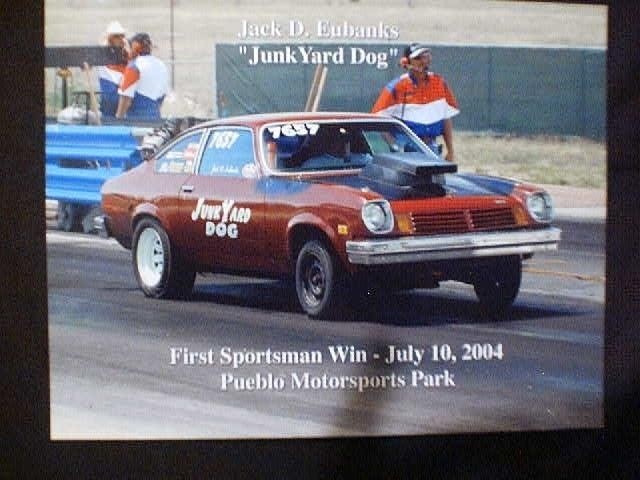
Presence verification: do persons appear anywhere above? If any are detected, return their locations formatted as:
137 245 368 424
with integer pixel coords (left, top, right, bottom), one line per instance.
113 33 169 123
300 128 373 169
369 43 460 164
96 21 133 124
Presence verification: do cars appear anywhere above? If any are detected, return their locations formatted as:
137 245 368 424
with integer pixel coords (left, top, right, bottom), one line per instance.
101 111 563 319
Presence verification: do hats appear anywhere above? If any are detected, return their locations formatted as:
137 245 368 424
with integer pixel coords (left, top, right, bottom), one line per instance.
403 42 432 59
107 22 151 45
